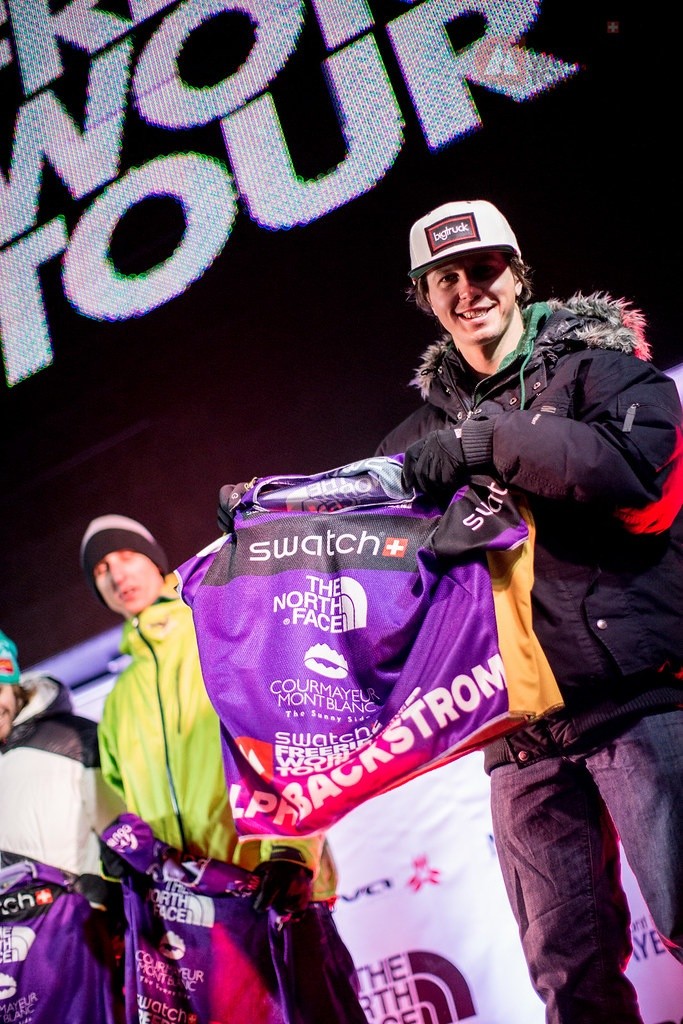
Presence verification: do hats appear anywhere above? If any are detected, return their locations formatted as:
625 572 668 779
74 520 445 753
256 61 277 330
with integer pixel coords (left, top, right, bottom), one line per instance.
79 514 169 609
407 200 524 286
0 631 20 684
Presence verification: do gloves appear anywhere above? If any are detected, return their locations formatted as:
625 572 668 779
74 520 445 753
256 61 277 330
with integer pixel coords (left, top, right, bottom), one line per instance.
248 854 314 927
76 874 123 915
402 427 466 494
216 478 256 534
98 840 132 879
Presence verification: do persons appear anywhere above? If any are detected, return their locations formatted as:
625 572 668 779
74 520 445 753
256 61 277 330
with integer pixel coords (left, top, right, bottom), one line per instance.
80 513 340 1024
0 625 104 1024
221 194 683 1022
